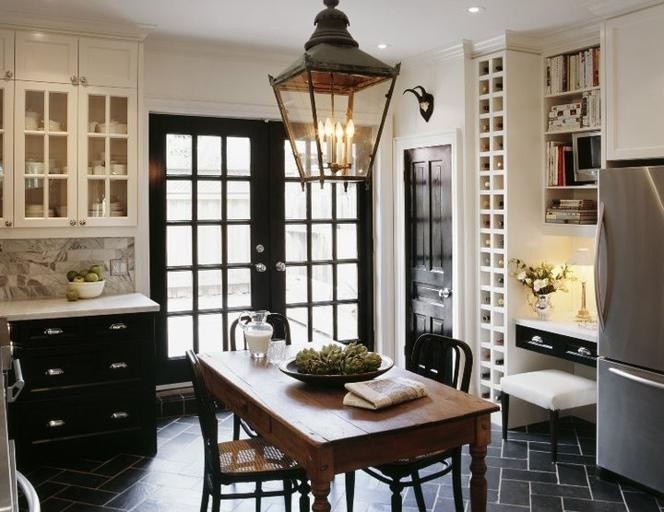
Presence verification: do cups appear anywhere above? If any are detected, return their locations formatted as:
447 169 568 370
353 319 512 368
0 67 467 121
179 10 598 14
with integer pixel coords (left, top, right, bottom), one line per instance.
265 336 287 365
25 111 127 174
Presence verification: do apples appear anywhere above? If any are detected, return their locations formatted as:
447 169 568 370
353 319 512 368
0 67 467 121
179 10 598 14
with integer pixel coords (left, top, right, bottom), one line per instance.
66 288 78 301
66 264 103 281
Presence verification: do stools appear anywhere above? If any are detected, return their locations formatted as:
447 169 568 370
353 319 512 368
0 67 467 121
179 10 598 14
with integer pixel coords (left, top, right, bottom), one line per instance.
499 366 605 464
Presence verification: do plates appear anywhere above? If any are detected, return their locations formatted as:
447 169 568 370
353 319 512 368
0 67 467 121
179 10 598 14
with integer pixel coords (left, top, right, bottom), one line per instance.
26 201 123 218
277 352 394 383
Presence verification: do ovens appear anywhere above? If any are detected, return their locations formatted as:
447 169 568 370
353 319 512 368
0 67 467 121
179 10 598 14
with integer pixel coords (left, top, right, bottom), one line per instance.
0 358 26 511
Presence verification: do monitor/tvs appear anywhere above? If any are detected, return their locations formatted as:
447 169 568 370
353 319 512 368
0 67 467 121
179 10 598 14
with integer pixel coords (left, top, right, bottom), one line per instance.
571 131 602 182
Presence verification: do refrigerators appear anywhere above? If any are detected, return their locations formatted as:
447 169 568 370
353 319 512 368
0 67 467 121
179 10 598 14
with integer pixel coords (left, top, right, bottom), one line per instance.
589 164 664 497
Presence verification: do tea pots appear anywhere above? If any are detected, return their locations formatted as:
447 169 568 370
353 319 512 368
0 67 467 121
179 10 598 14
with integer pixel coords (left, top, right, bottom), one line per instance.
236 310 274 360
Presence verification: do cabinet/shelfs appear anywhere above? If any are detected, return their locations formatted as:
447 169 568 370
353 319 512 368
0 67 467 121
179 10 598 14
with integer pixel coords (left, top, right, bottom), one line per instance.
601 5 664 162
0 22 137 241
12 313 157 467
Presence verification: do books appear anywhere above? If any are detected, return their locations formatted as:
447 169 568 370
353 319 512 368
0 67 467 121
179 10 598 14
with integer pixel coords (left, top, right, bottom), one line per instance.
541 44 600 225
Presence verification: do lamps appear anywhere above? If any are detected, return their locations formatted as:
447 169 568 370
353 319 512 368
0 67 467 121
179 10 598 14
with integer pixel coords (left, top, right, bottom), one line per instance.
266 0 400 189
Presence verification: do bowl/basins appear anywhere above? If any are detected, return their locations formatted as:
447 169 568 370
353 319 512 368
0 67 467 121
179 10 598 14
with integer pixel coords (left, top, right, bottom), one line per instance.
68 280 105 300
